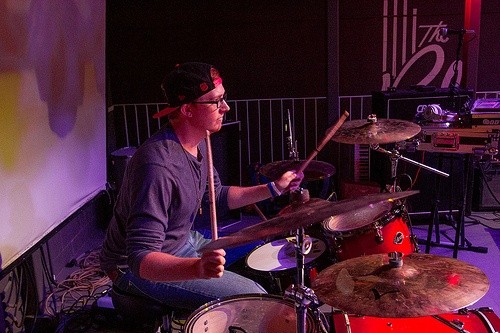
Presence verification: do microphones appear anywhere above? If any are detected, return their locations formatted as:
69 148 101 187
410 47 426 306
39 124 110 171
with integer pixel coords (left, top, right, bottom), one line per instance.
439 27 474 35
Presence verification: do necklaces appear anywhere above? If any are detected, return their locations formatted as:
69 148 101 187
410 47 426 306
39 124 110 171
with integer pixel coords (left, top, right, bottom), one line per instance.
182 146 202 214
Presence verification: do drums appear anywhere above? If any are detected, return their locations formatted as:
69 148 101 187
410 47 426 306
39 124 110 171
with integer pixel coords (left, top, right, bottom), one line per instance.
242 234 336 295
108 145 139 189
330 305 500 333
182 293 319 333
319 199 420 262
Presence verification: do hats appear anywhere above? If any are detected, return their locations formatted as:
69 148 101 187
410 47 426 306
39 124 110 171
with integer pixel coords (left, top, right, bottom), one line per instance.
152 62 223 119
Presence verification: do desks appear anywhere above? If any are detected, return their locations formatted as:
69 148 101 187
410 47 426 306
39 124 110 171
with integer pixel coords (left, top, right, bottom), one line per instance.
421 127 500 214
414 144 491 259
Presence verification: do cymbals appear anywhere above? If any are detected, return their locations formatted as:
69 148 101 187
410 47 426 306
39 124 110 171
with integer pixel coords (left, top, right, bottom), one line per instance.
325 117 422 146
312 253 491 319
194 188 422 255
258 158 339 184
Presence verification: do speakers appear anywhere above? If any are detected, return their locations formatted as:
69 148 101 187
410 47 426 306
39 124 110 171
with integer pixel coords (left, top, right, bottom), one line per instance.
191 122 243 231
370 87 476 224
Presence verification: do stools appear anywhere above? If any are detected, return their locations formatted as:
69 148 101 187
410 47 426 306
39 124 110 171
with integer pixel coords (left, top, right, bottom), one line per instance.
112 284 173 333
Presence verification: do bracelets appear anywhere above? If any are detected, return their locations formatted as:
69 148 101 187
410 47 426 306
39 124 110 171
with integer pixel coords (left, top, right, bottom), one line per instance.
268 183 276 197
271 182 281 196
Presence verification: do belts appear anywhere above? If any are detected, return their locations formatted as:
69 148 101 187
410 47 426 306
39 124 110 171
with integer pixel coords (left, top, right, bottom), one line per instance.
109 269 118 283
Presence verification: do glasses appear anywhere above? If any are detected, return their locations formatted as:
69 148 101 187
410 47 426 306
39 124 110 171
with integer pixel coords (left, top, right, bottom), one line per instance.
186 92 227 108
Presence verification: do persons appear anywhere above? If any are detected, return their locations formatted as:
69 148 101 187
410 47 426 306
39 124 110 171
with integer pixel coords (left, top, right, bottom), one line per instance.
100 63 304 311
